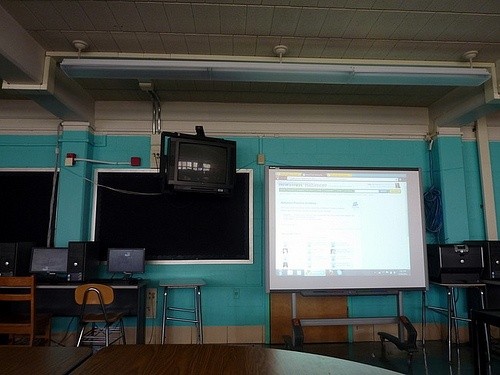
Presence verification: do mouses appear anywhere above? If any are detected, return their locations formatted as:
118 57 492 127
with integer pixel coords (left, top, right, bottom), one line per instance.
129 280 138 285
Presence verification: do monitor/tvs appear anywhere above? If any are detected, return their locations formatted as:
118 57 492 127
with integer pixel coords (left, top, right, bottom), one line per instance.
107 248 145 281
29 247 69 274
166 138 233 188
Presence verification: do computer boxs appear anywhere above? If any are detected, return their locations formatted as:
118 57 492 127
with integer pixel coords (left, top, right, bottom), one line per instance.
66 241 99 282
0 242 34 277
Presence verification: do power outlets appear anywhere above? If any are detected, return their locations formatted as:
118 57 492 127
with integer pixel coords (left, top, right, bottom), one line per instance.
145 288 157 319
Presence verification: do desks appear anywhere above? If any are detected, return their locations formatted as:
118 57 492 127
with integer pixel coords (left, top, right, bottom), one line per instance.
0 280 149 346
159 277 206 350
0 347 403 375
422 280 485 365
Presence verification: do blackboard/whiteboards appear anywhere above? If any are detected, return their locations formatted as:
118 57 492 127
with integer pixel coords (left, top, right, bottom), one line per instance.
0 168 60 253
91 168 254 264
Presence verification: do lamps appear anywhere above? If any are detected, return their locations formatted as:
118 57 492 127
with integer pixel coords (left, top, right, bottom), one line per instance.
59 57 490 86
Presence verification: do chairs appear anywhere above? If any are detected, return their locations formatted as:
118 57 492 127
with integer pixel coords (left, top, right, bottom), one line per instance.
0 274 52 346
74 284 126 348
